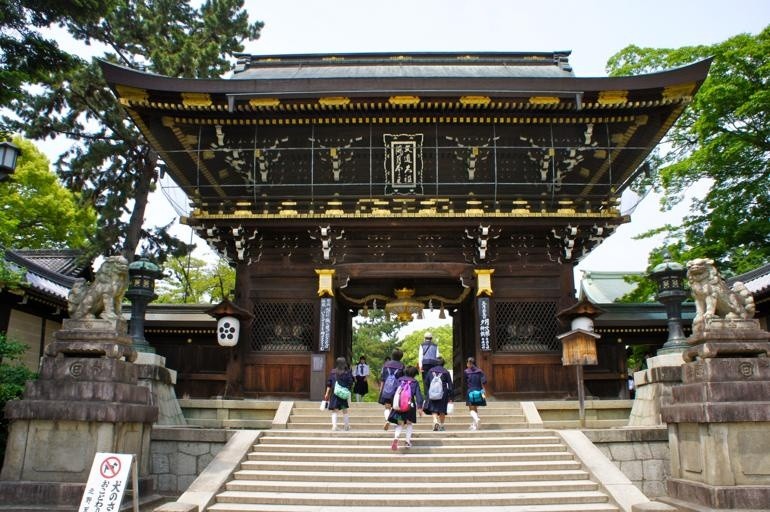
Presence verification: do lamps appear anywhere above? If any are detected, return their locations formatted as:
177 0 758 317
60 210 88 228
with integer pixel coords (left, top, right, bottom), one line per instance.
123 256 165 353
647 253 691 354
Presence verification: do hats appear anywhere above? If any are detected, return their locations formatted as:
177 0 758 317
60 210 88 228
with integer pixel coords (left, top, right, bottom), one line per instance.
425 332 433 338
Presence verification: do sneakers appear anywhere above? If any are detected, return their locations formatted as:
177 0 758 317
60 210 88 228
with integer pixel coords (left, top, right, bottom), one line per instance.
433 423 445 431
384 421 389 430
405 442 412 446
468 418 481 430
392 439 398 450
332 426 350 432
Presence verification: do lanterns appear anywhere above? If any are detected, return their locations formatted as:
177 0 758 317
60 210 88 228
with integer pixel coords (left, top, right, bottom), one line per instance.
216 316 241 347
314 267 337 296
570 317 595 333
473 268 496 297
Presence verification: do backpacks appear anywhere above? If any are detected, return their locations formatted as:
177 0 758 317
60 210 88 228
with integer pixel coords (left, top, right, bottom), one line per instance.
428 372 444 400
382 368 399 399
393 380 414 411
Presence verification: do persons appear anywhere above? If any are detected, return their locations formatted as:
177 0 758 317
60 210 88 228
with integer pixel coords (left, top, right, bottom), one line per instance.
380 357 391 375
354 355 371 403
419 333 440 398
390 364 423 450
325 356 354 432
423 357 454 432
464 357 489 431
381 349 405 432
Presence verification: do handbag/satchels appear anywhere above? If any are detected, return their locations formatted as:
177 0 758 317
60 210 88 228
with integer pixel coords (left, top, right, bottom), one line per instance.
469 391 483 402
334 381 350 400
